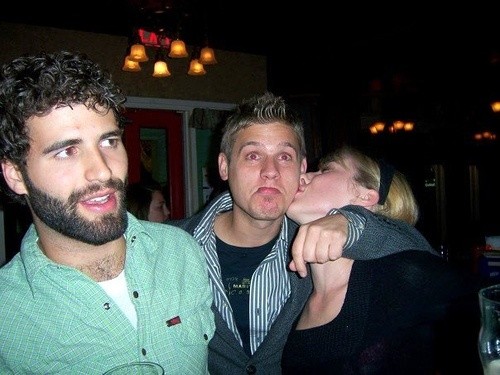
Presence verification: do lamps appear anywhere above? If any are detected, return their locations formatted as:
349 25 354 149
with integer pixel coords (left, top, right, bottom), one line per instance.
122 28 219 78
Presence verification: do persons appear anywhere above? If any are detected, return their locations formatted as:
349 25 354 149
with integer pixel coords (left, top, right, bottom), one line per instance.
154 90 434 375
124 182 170 224
1 54 215 372
279 144 494 375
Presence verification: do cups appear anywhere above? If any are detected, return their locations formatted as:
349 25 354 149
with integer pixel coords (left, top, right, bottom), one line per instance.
102 361 165 375
436 246 449 262
478 284 500 375
